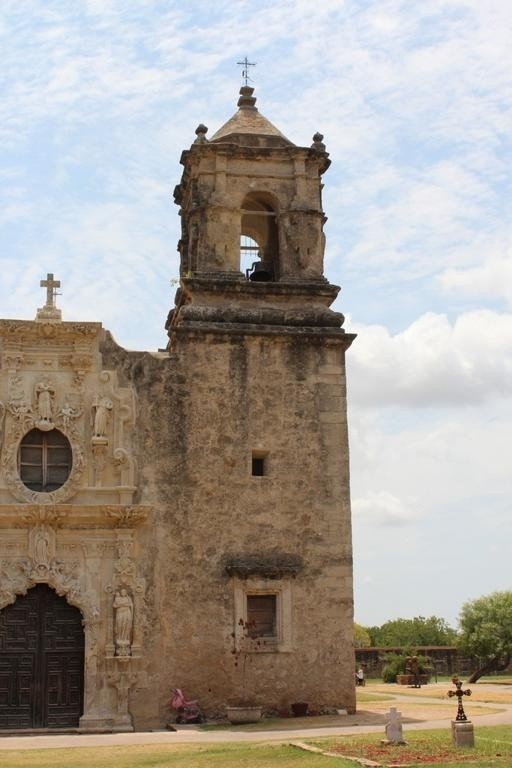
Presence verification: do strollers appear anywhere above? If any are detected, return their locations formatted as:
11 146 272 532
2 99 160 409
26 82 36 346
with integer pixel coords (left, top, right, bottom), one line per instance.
168 687 205 723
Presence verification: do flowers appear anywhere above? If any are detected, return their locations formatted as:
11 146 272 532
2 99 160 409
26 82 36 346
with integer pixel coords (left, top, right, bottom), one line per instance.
224 617 267 705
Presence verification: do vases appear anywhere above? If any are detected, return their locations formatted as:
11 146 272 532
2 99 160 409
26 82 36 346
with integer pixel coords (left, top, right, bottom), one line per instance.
226 705 262 725
